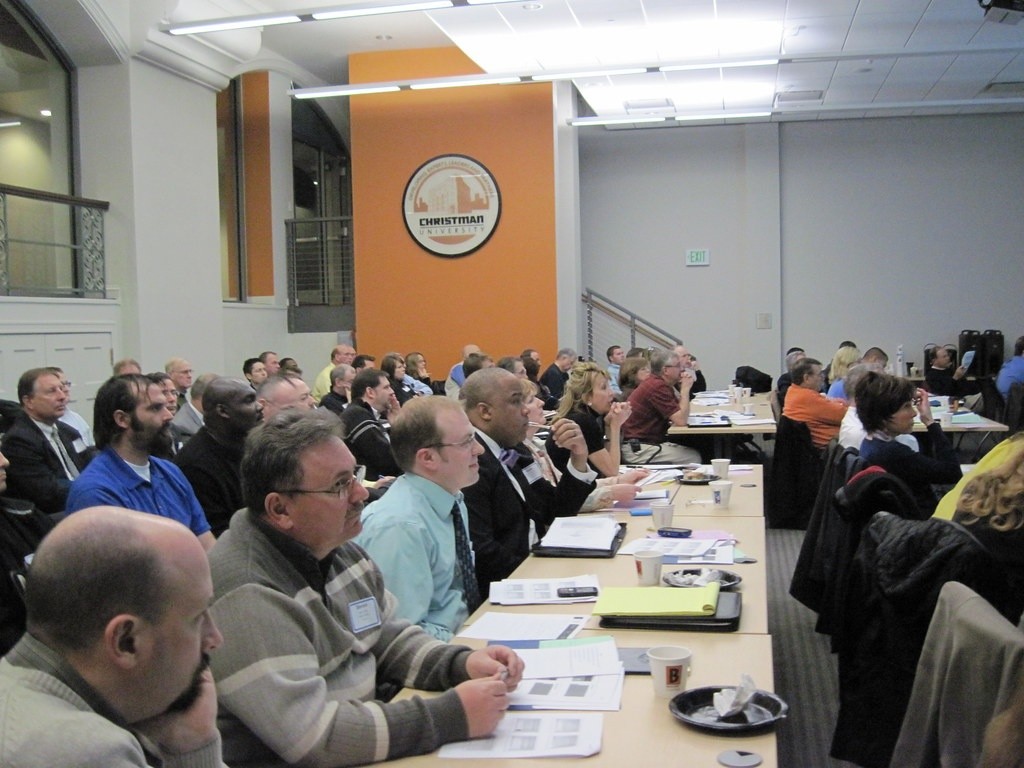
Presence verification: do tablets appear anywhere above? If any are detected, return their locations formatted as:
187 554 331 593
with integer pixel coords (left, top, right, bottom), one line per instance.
598 592 743 631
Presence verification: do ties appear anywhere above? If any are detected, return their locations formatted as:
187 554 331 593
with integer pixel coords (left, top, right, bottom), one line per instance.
451 500 480 615
51 427 80 479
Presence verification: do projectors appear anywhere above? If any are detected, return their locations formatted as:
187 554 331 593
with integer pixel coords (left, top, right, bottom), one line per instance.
984 0 1024 25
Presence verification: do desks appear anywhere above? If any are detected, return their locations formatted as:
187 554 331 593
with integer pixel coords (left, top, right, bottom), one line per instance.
911 396 1010 463
462 517 769 634
904 375 998 387
667 386 778 464
595 464 764 517
356 613 776 768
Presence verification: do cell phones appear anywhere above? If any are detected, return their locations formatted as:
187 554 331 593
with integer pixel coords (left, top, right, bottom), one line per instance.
658 527 691 537
557 586 598 596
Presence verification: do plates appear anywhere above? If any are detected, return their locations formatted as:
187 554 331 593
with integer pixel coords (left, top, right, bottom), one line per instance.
662 568 742 589
668 686 789 732
673 474 721 484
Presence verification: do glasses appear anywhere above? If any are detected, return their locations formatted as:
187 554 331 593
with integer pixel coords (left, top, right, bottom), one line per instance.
271 465 367 499
62 382 71 386
663 364 681 368
417 430 475 450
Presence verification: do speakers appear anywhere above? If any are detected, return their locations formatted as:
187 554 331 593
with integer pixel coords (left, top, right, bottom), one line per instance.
959 330 983 374
983 330 1004 374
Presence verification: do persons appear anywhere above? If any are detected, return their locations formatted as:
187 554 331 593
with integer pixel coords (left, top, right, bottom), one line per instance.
456 365 600 600
351 394 486 642
543 360 633 478
0 339 1024 622
0 505 229 768
63 373 217 555
207 403 525 768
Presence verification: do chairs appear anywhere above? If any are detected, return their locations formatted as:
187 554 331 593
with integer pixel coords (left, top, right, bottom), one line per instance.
828 509 1024 768
808 447 928 654
765 414 823 531
770 388 781 422
955 375 1007 463
786 437 847 613
997 383 1024 442
890 579 1024 768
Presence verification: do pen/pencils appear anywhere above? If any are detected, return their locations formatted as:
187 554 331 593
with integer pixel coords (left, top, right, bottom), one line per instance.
696 415 712 417
528 422 551 429
626 466 642 468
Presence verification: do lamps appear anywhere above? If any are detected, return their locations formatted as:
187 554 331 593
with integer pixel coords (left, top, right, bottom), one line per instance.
155 0 1024 128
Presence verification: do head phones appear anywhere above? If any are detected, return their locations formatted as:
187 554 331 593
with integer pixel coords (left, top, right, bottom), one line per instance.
929 346 937 359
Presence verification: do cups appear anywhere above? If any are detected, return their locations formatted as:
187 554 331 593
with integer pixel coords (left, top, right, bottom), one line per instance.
708 480 733 509
948 396 958 412
728 384 752 401
710 459 731 478
941 412 953 426
905 361 919 377
650 502 676 529
743 403 753 414
648 645 693 696
634 550 663 585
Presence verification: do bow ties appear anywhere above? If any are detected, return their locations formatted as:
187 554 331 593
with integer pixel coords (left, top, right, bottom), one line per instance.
498 449 519 469
